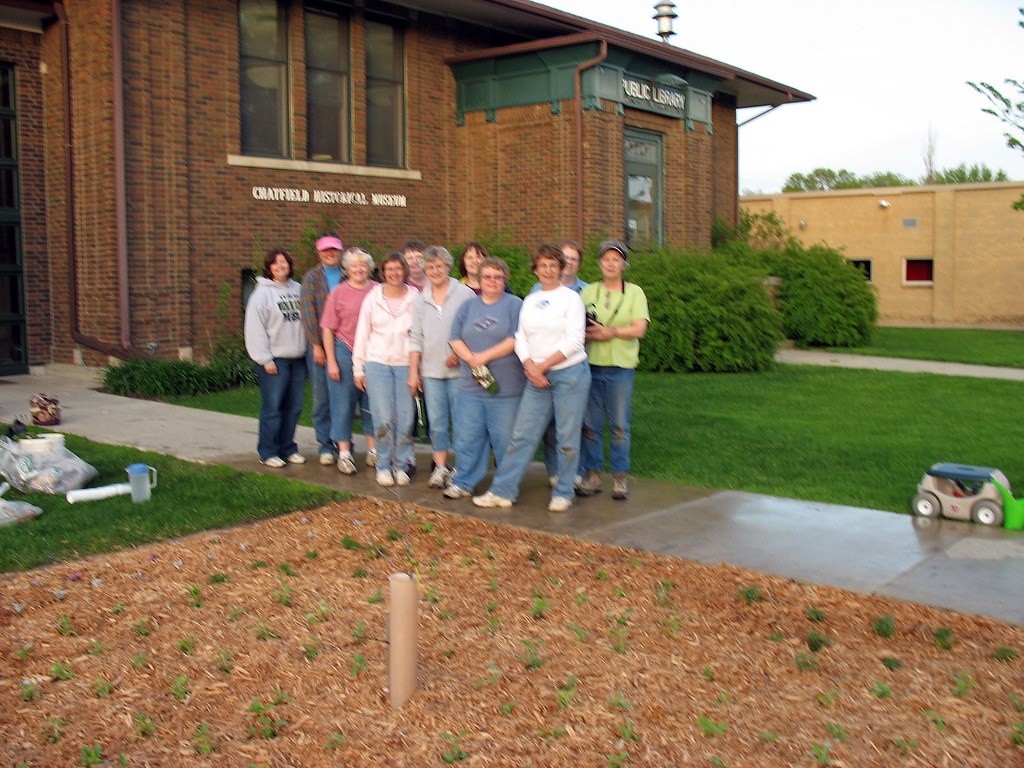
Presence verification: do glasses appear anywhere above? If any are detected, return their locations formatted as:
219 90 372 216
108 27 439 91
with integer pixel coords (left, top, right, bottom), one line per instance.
349 247 368 254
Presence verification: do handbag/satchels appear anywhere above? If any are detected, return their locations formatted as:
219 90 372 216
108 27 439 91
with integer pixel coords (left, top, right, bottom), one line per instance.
29 392 61 424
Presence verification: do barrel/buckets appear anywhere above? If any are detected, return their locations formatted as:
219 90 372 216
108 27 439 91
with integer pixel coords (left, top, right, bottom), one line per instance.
19 432 65 454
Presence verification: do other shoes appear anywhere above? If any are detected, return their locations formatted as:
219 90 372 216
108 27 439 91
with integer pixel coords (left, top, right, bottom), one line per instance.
445 467 457 487
428 466 449 488
441 485 472 498
611 473 628 499
574 474 582 489
549 475 558 487
575 471 603 496
408 456 417 476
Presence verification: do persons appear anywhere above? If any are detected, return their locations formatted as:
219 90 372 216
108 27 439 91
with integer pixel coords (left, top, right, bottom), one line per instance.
243 248 307 468
301 230 651 512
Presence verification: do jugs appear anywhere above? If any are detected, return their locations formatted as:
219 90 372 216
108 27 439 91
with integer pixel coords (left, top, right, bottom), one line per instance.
125 463 157 502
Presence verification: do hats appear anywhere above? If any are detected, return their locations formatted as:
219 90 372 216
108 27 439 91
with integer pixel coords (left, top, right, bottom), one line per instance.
316 236 343 251
598 240 628 261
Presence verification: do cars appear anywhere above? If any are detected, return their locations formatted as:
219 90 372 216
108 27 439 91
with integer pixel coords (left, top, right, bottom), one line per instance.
912 461 1013 528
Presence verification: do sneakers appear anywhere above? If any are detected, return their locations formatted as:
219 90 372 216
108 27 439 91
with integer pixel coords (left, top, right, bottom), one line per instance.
337 454 357 474
320 453 334 465
472 490 512 507
365 450 377 467
548 496 572 511
259 456 287 467
393 470 410 486
286 452 307 463
376 466 394 486
